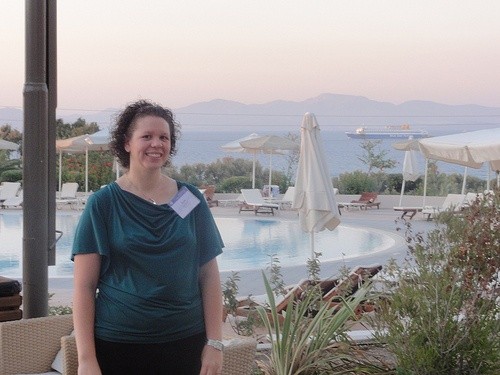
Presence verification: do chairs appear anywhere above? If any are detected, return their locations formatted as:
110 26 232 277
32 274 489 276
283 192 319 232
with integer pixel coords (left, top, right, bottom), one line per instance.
238 185 338 216
0 181 80 209
201 184 219 208
222 262 391 326
339 193 381 211
394 193 483 223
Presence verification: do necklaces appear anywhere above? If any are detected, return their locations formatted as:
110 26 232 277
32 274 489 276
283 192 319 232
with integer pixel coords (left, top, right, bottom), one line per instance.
125 170 164 206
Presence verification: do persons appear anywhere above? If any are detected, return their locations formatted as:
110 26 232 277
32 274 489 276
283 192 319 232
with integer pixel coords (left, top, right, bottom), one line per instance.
71 101 225 375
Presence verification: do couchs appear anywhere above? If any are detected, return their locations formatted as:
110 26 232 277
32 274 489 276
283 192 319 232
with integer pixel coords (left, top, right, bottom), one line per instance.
0 314 258 375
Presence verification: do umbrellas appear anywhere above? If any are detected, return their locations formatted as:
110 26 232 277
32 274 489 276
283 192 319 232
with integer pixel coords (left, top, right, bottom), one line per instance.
291 112 342 280
220 133 300 200
0 139 21 151
394 126 500 206
56 129 120 200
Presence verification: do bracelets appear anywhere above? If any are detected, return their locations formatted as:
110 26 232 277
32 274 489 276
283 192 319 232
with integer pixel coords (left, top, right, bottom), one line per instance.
207 338 224 351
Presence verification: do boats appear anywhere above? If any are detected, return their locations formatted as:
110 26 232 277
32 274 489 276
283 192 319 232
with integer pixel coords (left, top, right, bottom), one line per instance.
345 130 430 140
